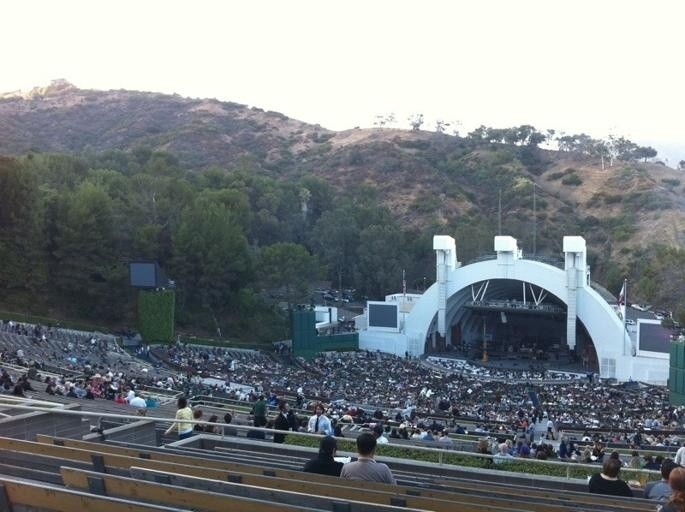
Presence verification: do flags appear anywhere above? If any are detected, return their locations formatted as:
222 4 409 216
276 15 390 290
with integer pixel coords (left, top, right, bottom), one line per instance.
618 282 626 304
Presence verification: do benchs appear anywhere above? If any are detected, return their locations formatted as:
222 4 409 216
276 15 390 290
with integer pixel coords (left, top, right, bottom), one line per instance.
0 434 669 512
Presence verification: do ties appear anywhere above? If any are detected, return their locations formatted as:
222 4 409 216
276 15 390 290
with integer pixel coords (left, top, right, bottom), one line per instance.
315 418 319 432
